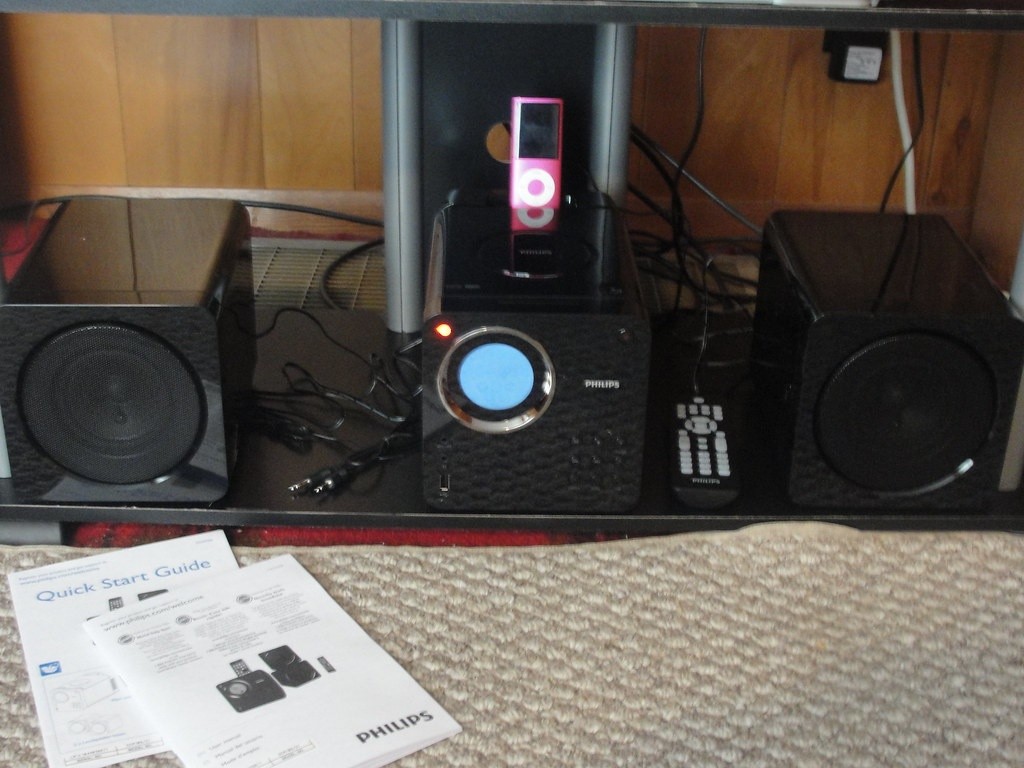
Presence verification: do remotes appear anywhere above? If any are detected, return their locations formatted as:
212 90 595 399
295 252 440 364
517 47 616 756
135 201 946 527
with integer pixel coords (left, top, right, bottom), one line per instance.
669 391 738 510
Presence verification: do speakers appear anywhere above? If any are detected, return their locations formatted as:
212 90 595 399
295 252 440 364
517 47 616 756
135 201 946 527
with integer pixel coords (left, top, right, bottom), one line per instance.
749 210 1024 515
0 194 257 505
419 205 653 515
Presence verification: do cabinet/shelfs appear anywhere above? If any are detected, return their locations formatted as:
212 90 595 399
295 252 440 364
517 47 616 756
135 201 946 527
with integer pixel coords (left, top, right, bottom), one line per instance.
1 0 1024 545
